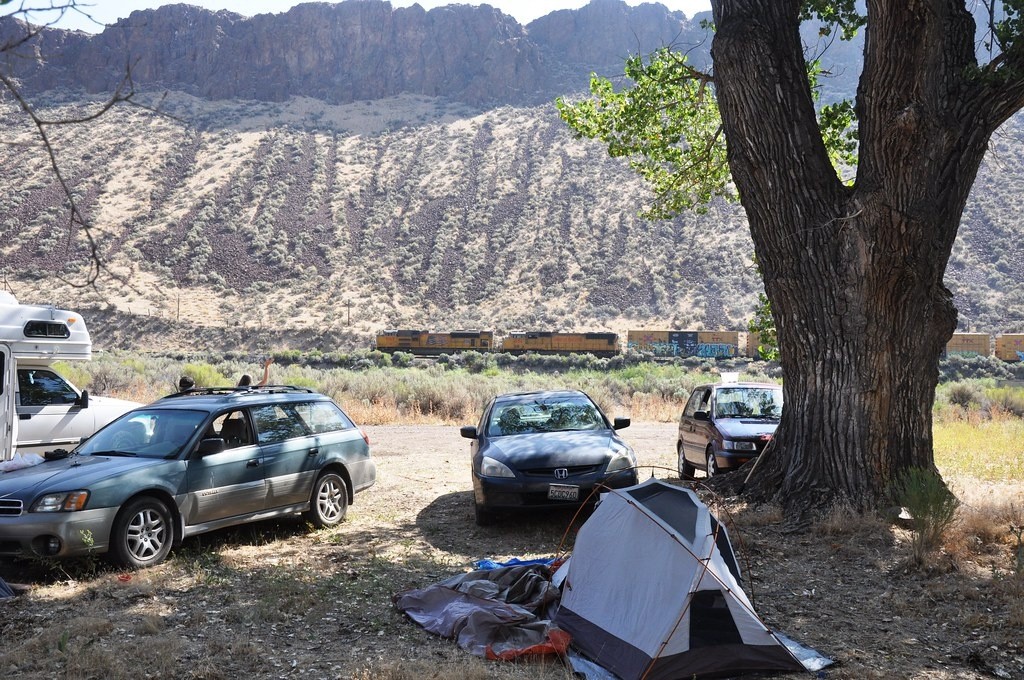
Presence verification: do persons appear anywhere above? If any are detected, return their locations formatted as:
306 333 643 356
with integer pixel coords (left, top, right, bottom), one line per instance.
178 376 195 394
236 358 273 389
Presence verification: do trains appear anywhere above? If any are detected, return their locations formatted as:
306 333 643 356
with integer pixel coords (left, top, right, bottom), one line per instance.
374 327 1024 363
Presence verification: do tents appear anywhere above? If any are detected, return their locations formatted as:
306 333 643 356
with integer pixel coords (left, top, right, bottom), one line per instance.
558 475 834 680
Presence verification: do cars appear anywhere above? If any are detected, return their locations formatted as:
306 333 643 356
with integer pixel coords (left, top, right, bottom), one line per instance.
0 384 378 573
460 389 641 526
678 378 783 481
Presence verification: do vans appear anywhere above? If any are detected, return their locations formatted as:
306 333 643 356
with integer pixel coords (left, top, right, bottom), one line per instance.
1 290 158 464
